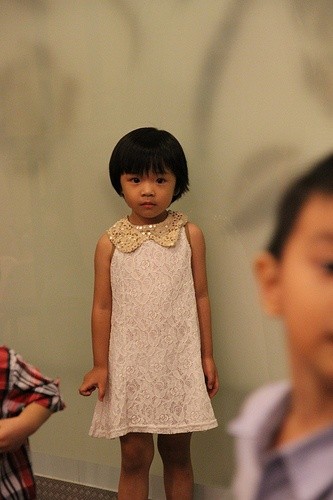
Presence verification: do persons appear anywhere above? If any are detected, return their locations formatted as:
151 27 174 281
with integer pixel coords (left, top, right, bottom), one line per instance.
221 152 333 499
78 128 221 500
0 347 65 499
